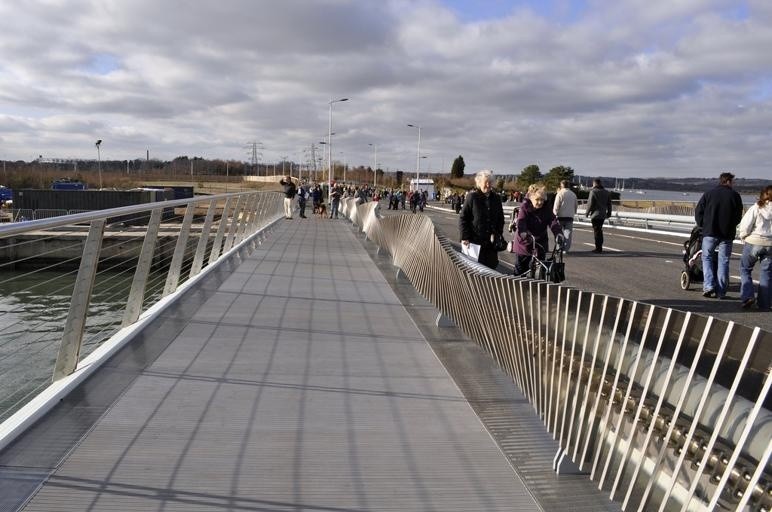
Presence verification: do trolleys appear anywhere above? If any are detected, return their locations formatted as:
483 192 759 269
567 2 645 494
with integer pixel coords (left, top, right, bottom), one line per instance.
515 232 567 284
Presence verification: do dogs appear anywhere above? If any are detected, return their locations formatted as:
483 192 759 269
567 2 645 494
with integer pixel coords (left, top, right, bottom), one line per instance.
318 204 328 219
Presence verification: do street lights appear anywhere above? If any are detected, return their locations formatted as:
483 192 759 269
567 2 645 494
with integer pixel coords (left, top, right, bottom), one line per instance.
409 124 428 193
319 141 336 179
340 151 347 182
323 132 336 180
328 96 353 207
95 140 102 190
366 143 384 187
250 149 310 181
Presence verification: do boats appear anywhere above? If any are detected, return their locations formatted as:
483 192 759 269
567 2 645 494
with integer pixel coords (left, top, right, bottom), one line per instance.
628 190 634 192
681 192 689 197
613 177 625 192
636 191 646 195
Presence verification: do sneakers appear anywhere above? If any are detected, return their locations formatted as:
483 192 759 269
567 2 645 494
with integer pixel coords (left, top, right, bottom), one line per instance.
741 299 754 309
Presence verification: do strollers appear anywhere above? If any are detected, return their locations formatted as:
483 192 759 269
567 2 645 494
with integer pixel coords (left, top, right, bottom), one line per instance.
679 225 719 291
505 206 519 252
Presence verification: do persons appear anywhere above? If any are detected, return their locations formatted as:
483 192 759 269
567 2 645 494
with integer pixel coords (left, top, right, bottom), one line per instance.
460 171 505 271
739 185 772 311
512 187 565 278
586 179 612 252
553 180 578 252
694 173 742 299
279 176 524 221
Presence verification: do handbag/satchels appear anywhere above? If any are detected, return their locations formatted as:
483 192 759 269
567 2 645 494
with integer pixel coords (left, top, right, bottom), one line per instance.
551 263 565 283
493 232 507 251
